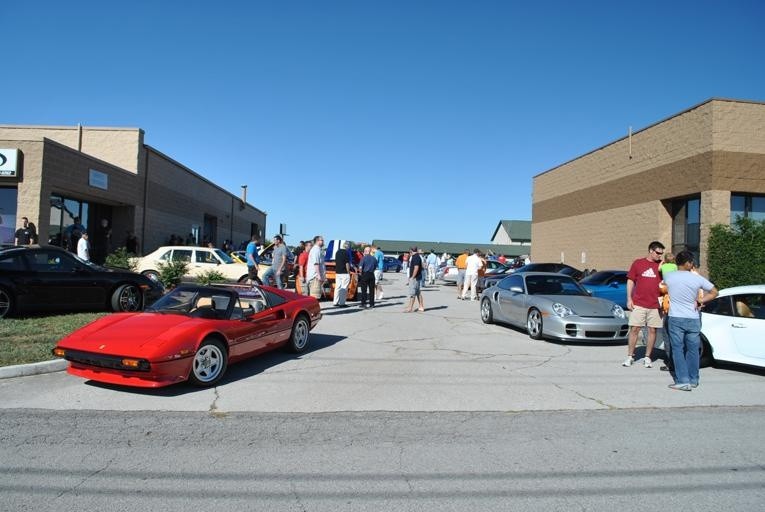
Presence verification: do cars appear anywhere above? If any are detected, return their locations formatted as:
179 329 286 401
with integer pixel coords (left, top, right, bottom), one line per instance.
52 281 322 388
641 284 765 368
436 254 631 311
127 243 274 286
0 243 164 320
480 272 631 342
295 260 358 301
383 257 401 272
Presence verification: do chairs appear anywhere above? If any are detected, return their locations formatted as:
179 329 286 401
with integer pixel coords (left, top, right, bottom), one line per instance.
188 298 215 314
228 301 252 321
736 296 755 317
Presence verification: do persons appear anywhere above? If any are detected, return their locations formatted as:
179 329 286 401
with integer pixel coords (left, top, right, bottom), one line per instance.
658 250 719 391
400 245 508 313
14 217 36 246
524 256 531 265
659 262 704 371
168 235 385 310
116 226 141 257
622 241 666 368
658 253 678 279
65 217 113 263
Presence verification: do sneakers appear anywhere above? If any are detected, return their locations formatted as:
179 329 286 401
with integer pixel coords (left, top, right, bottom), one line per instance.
622 356 633 367
669 383 698 391
660 366 674 371
643 358 652 368
